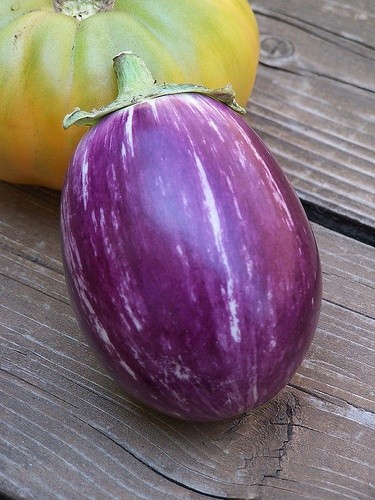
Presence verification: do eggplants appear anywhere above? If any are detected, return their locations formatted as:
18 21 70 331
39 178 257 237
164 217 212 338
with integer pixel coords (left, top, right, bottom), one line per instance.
61 51 322 424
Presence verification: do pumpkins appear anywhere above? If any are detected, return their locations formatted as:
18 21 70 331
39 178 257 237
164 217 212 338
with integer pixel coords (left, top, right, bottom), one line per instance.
0 0 261 192
